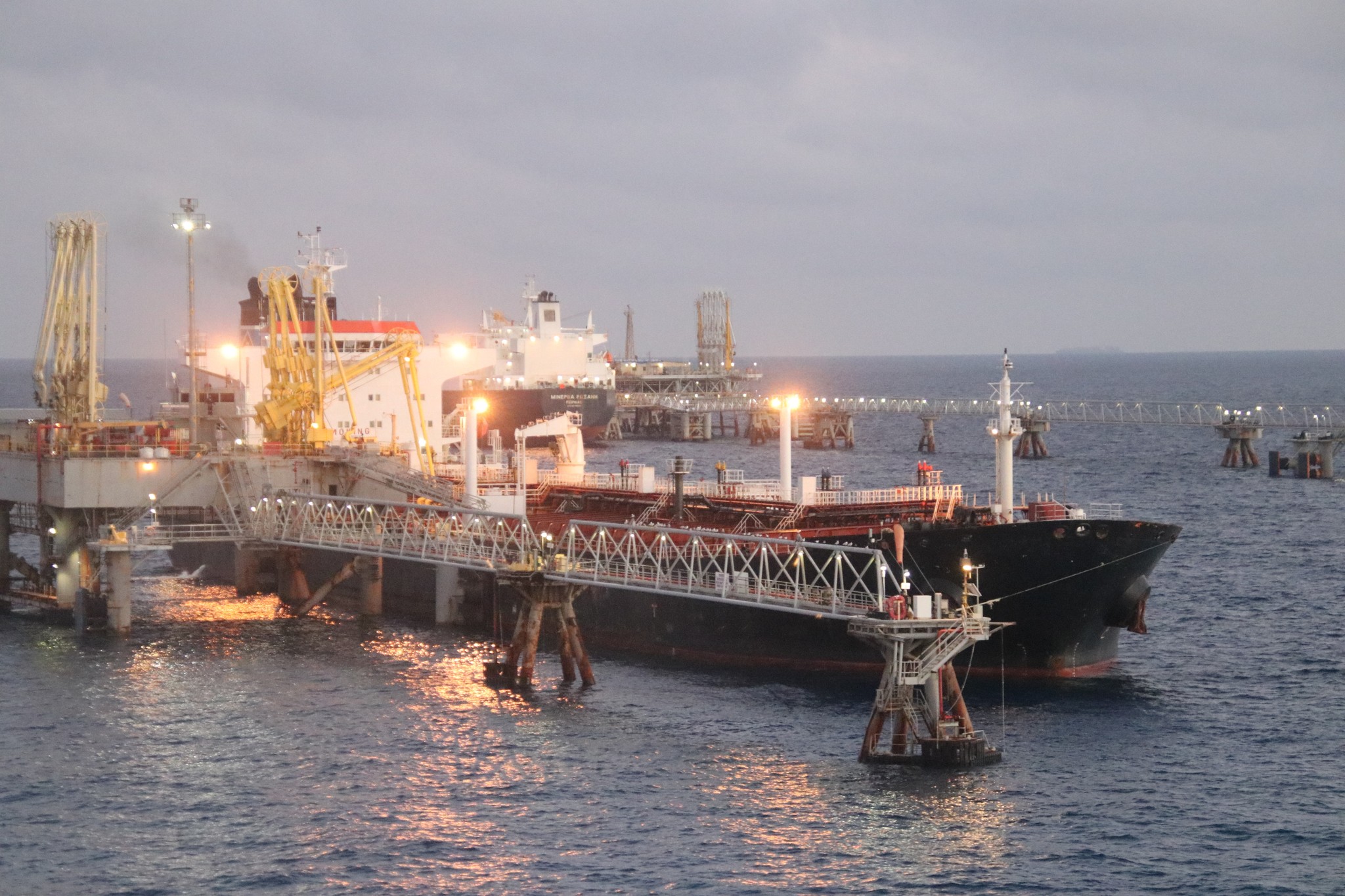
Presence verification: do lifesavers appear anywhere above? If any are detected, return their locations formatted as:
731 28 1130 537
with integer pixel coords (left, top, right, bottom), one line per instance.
888 595 907 620
204 383 212 391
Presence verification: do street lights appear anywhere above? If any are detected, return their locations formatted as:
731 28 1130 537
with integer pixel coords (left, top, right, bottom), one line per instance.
171 219 212 457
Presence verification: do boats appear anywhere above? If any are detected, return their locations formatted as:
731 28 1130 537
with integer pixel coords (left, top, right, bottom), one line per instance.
438 288 623 450
425 346 1184 686
